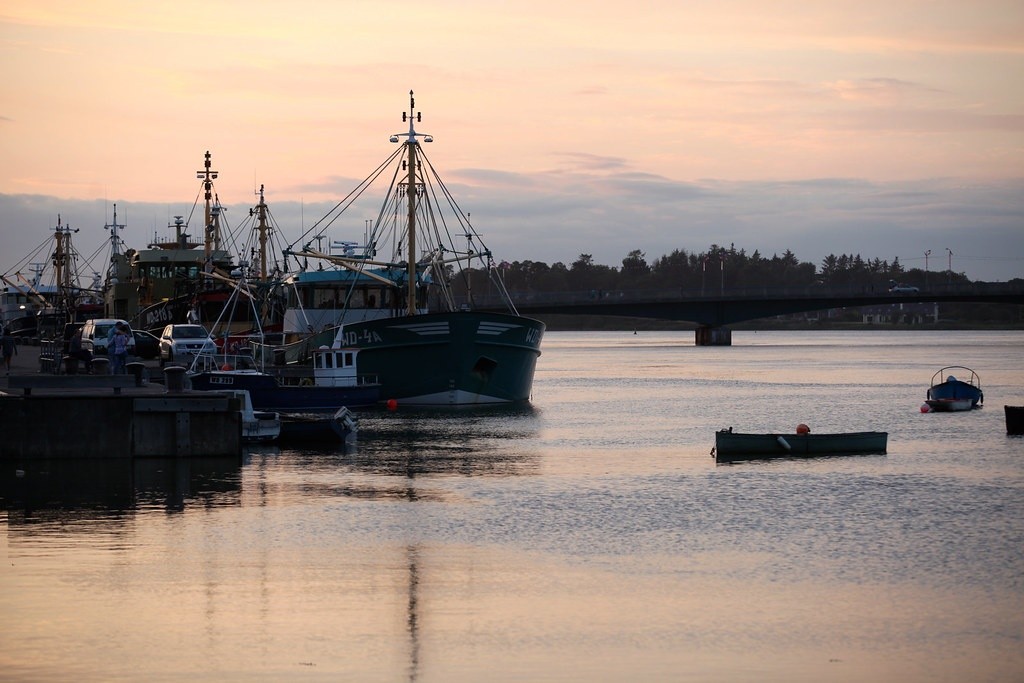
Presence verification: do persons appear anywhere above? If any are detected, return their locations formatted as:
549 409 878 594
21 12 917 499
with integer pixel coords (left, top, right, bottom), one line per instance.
107 321 130 374
0 328 17 375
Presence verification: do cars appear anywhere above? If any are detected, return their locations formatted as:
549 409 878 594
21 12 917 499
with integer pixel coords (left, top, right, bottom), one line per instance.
132 328 161 359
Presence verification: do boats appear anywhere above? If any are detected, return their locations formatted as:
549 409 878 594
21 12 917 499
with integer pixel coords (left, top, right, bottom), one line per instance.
0 88 546 459
711 423 888 464
1003 405 1024 436
694 324 733 347
923 366 984 412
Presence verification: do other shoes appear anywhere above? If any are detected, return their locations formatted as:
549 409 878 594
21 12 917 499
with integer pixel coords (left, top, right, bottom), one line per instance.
88 371 94 375
5 372 9 375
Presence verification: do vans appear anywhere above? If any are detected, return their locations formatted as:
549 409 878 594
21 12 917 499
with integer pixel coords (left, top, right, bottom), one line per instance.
80 317 136 357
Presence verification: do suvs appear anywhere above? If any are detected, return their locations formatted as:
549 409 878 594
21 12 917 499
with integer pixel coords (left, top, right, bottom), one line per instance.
158 323 219 367
